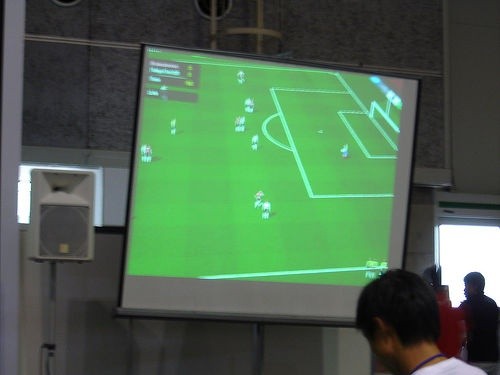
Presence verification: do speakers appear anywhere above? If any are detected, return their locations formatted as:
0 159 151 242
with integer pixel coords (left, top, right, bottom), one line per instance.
27 168 95 263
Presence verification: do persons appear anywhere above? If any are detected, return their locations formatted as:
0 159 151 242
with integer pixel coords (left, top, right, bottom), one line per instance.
457 272 500 367
353 268 488 375
142 68 389 280
427 283 468 360
